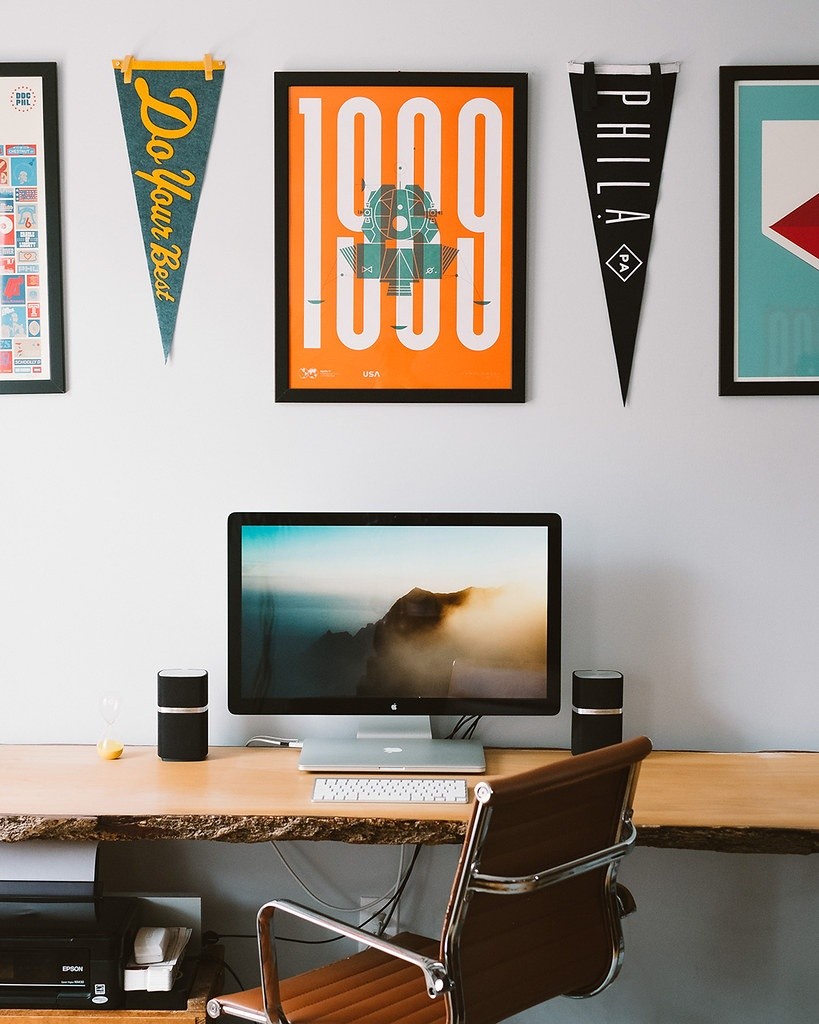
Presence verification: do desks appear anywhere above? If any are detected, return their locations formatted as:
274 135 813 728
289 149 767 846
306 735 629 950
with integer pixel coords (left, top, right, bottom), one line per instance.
0 743 819 856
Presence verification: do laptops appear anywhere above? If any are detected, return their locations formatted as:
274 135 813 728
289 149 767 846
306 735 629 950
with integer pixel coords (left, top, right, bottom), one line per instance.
300 739 486 773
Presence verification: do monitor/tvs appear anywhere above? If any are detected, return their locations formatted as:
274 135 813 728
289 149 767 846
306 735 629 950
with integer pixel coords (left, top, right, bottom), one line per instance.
227 512 562 739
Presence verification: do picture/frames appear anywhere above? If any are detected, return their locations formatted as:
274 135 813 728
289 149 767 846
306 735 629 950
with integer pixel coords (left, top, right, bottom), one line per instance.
276 72 527 404
0 62 66 396
721 65 819 397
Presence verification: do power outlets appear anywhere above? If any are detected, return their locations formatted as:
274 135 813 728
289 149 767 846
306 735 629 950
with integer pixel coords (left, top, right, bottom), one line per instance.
358 897 400 953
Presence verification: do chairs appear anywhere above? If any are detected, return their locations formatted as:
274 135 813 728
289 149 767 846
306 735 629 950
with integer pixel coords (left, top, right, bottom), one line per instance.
217 736 654 1024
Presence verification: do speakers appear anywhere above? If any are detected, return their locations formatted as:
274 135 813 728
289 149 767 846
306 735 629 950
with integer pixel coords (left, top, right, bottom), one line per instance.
157 669 209 759
572 669 623 755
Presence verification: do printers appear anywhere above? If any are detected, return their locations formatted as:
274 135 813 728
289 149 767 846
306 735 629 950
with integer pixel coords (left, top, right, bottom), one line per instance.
0 881 141 1011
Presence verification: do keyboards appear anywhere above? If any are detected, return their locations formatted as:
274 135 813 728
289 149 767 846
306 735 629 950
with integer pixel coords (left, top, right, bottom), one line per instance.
313 778 469 804
